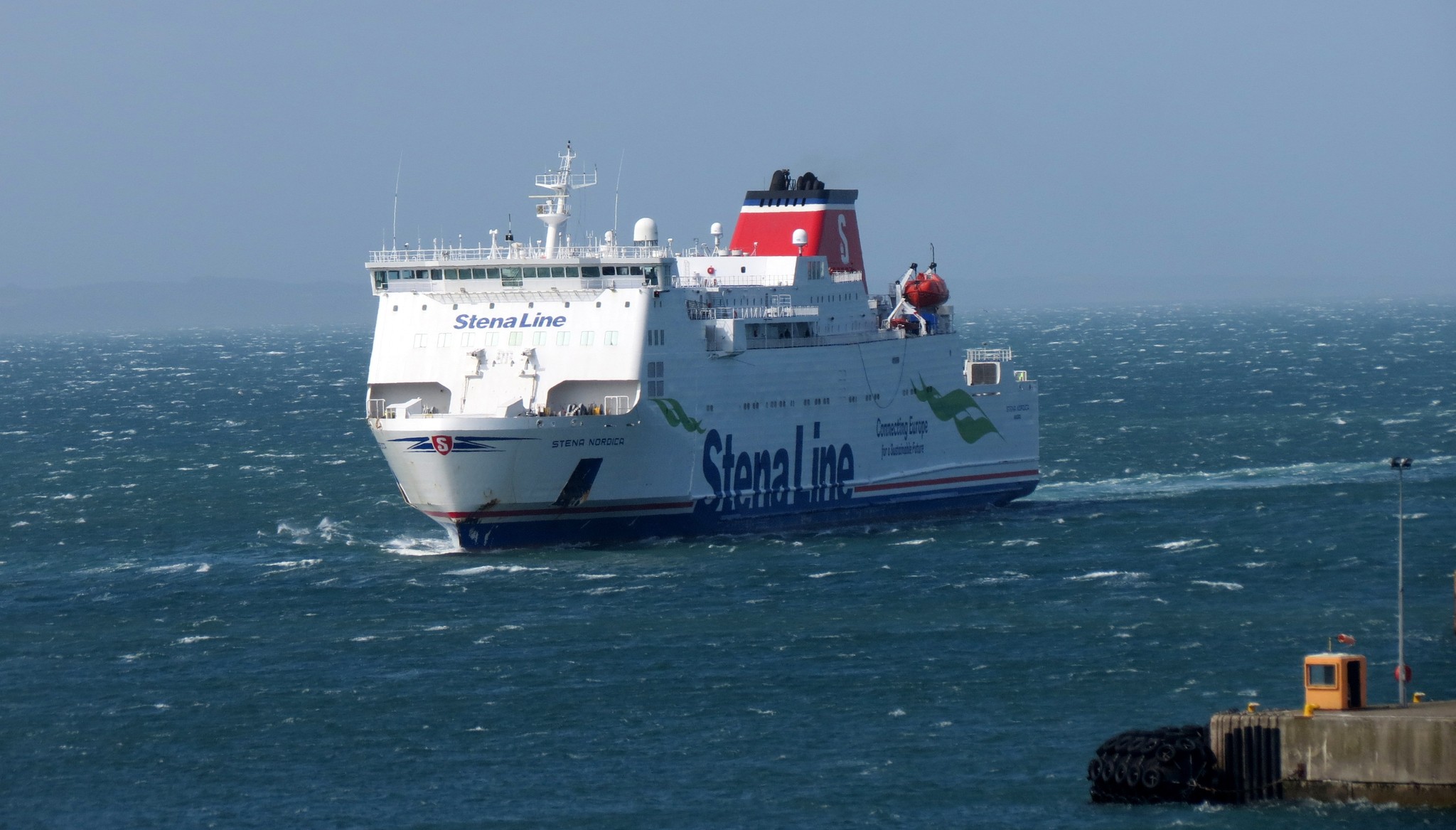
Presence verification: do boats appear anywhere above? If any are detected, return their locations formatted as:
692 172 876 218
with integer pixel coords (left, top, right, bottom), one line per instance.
891 316 917 331
364 137 1041 548
904 272 950 309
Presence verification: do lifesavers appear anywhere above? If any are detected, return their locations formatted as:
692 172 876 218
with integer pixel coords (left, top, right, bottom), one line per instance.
1087 725 1225 806
1394 663 1414 683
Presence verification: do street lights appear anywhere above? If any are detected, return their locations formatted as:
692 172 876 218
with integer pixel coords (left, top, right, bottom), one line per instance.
1387 457 1416 706
